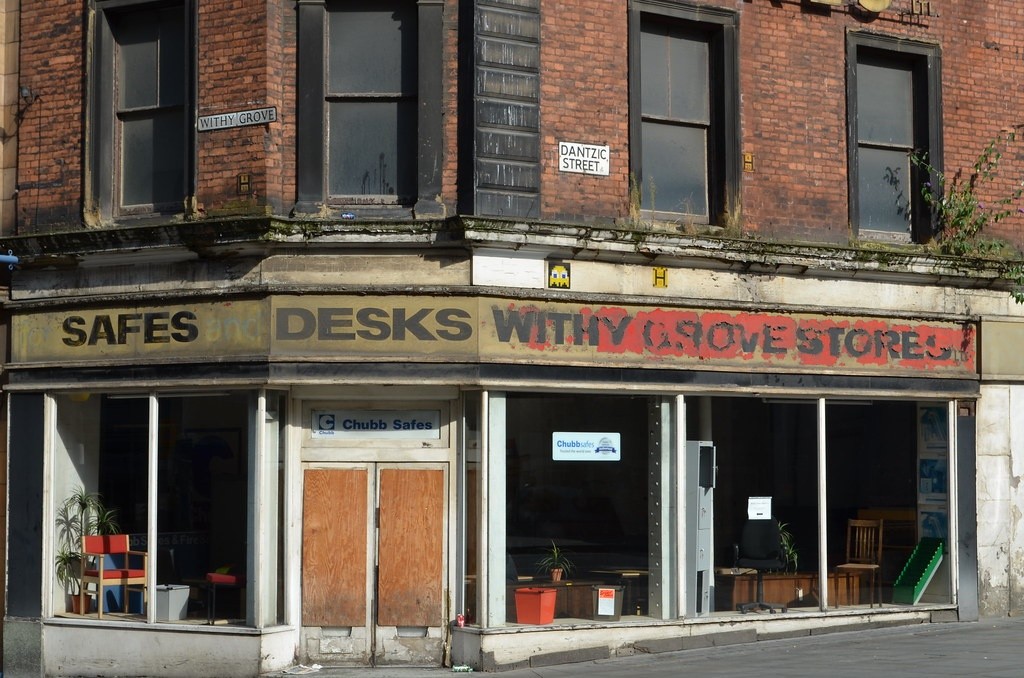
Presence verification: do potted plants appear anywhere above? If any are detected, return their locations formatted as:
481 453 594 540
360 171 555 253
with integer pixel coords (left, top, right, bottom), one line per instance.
55 487 122 614
535 538 575 582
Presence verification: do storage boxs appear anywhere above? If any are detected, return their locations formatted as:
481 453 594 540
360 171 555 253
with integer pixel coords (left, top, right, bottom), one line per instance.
591 585 625 621
156 584 190 622
513 588 558 625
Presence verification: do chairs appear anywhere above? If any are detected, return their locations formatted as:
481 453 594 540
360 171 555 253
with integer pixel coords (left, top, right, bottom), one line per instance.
731 520 787 614
80 534 148 619
206 573 246 626
834 519 884 608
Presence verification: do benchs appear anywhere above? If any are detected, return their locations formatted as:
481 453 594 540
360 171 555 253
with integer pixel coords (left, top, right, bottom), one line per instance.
858 508 917 549
